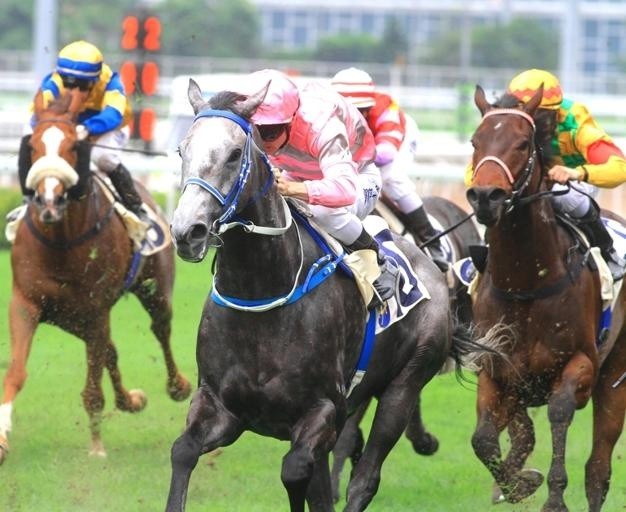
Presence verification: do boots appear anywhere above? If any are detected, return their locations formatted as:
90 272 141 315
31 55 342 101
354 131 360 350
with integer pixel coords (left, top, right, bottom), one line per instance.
109 163 154 231
407 205 448 271
571 200 613 254
348 226 400 309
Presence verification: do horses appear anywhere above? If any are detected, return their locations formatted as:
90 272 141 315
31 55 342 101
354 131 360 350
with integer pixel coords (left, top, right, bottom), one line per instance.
325 192 484 507
0 85 193 467
464 80 626 512
166 76 516 511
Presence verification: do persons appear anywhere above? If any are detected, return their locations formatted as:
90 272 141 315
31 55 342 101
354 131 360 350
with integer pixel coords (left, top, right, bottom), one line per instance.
325 65 452 273
465 68 626 340
229 68 403 304
4 39 151 243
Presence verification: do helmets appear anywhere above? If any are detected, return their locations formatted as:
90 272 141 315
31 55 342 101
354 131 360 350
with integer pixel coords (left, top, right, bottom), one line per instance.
58 42 104 79
240 69 301 125
508 68 562 110
332 68 376 108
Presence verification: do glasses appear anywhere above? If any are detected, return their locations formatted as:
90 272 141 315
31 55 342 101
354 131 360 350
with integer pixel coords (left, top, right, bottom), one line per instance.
255 125 288 141
59 73 94 90
